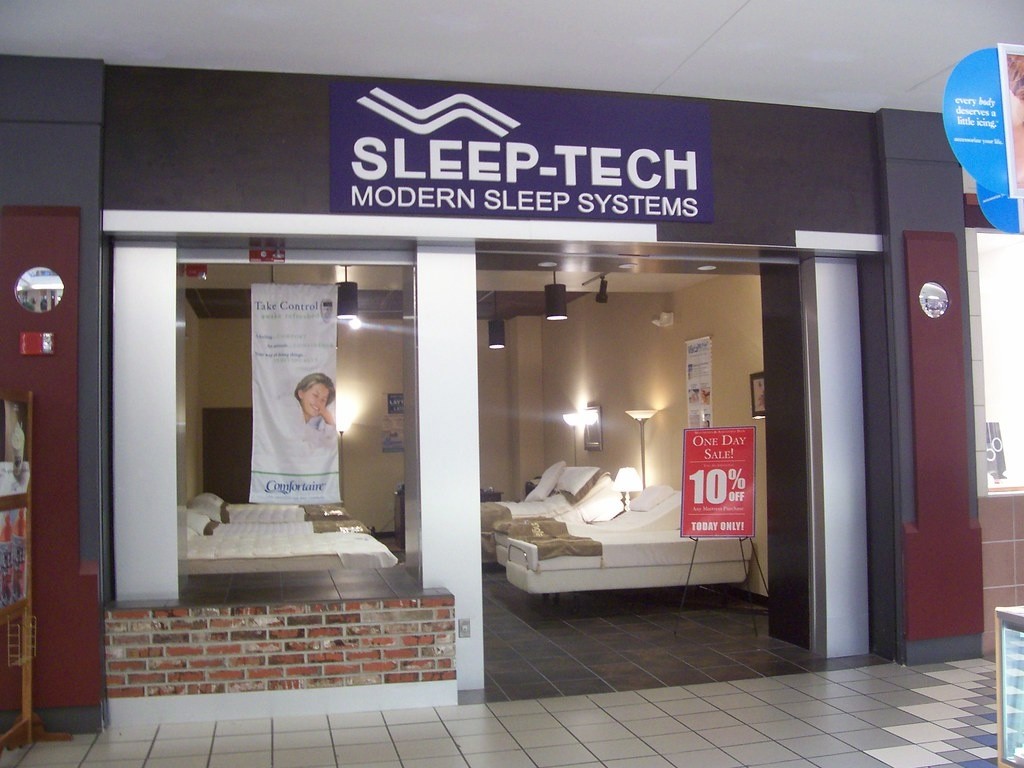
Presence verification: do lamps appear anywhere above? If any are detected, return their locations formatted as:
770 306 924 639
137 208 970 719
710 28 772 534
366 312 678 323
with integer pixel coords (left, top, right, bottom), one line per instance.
625 410 658 488
336 264 357 319
562 412 582 467
487 292 505 348
612 467 644 511
544 268 568 320
596 276 608 303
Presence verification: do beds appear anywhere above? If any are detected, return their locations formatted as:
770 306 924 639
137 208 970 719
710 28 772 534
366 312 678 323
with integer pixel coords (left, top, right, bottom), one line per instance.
480 460 752 616
187 493 397 575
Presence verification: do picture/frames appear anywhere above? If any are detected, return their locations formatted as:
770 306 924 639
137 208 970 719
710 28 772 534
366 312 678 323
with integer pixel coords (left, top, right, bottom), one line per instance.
749 372 765 418
582 404 603 451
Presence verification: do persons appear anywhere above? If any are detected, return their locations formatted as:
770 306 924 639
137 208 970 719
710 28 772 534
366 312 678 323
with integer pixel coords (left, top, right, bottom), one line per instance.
253 373 335 472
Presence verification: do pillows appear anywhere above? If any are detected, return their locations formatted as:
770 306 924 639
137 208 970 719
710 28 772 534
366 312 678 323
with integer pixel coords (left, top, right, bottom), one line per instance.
555 466 598 496
187 527 198 542
524 460 566 502
629 484 674 511
187 509 209 535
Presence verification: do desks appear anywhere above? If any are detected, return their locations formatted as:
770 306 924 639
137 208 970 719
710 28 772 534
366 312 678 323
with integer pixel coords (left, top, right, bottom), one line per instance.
393 489 504 554
